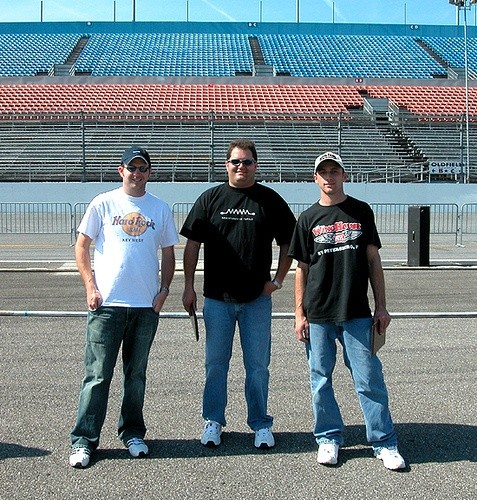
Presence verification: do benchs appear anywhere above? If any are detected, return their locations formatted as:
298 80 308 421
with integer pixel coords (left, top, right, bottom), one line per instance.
0 121 477 185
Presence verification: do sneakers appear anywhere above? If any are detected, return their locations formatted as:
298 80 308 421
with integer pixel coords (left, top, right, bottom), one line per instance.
126 437 149 457
374 446 406 469
254 426 275 448
69 447 91 467
200 420 223 446
317 444 339 465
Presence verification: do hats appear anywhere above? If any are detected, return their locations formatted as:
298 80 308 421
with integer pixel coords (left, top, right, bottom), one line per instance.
314 152 345 174
120 146 150 168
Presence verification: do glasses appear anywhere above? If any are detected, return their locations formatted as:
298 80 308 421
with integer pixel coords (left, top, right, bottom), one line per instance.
123 165 148 173
229 159 253 166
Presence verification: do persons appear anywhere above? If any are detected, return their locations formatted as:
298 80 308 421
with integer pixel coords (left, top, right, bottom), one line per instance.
288 152 406 471
68 146 180 468
179 138 297 449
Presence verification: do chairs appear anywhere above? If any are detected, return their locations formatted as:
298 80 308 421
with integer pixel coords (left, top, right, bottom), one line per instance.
0 33 477 123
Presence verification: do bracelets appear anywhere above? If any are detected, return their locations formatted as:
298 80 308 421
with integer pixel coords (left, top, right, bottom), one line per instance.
272 279 282 289
160 287 169 296
